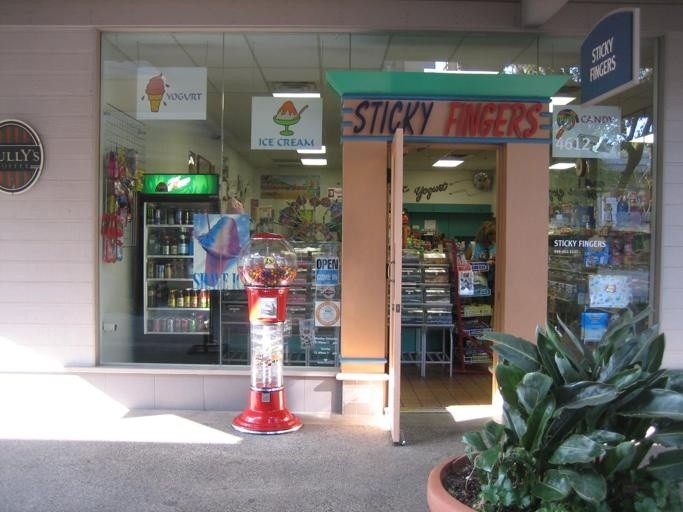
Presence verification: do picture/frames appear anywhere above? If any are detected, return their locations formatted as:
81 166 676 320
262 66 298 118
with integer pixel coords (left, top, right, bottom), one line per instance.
197 154 211 173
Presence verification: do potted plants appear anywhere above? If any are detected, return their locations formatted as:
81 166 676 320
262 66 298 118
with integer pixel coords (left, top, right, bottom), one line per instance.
427 301 683 512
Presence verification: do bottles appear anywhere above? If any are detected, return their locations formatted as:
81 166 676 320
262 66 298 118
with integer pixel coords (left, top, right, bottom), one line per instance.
143 203 213 333
582 236 643 270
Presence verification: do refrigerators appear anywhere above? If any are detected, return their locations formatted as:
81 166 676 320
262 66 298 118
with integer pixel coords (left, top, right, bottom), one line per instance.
133 173 220 364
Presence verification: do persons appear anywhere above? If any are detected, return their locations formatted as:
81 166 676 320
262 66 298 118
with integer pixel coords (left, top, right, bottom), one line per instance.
463 220 496 260
